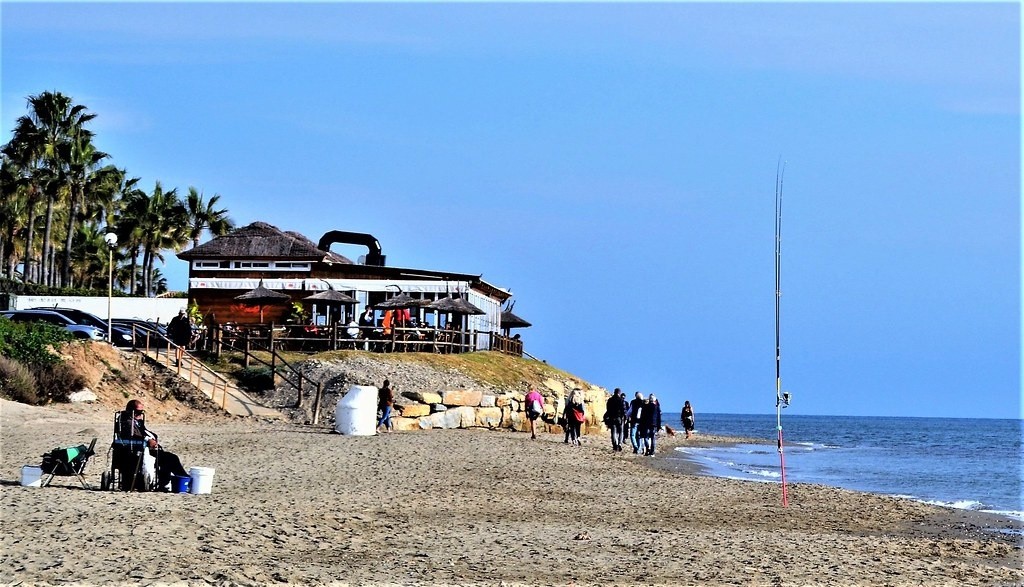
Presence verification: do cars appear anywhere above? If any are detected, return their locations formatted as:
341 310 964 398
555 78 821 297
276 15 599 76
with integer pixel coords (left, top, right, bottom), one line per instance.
22 306 171 348
0 311 107 345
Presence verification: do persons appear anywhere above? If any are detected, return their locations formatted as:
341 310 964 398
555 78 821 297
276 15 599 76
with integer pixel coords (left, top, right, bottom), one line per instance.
341 317 360 349
359 305 377 350
562 388 586 446
602 388 661 456
376 379 397 434
303 319 318 351
167 309 201 367
525 384 546 439
116 399 191 493
681 401 694 439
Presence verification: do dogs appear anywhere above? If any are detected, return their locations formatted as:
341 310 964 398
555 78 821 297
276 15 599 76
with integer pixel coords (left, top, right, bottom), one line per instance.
665 424 675 437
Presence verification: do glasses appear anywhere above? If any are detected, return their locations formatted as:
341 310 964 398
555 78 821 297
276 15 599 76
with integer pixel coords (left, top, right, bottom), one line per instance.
134 410 146 415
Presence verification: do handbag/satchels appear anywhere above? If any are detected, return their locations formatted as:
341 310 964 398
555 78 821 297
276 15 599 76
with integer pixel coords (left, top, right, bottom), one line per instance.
573 408 585 424
532 399 543 415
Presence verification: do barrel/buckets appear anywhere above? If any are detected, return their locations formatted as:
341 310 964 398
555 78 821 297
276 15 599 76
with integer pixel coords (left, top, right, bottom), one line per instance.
21 465 43 487
191 467 215 493
176 477 192 493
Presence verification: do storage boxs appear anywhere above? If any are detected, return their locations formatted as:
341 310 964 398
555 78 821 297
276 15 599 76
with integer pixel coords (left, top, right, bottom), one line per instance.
21 465 43 488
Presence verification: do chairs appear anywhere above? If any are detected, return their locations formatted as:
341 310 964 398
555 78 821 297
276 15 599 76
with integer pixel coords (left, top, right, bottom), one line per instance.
106 410 162 493
40 438 98 490
267 327 286 350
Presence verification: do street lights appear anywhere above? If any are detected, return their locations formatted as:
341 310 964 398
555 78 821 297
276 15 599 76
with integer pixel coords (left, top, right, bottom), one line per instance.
104 232 120 343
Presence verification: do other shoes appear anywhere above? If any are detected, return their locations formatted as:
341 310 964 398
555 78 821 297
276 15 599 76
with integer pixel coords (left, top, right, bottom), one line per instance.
531 435 537 440
633 447 638 454
618 444 622 452
645 449 654 456
572 440 575 445
176 358 184 367
577 437 581 446
613 447 617 450
564 440 568 445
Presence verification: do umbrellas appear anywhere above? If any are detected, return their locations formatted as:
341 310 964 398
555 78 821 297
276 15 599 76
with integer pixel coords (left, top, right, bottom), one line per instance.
234 281 291 323
501 312 532 338
373 292 431 327
421 297 486 332
302 286 360 326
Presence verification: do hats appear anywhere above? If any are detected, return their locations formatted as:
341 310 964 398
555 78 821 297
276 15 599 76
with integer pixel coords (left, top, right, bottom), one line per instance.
178 309 186 314
